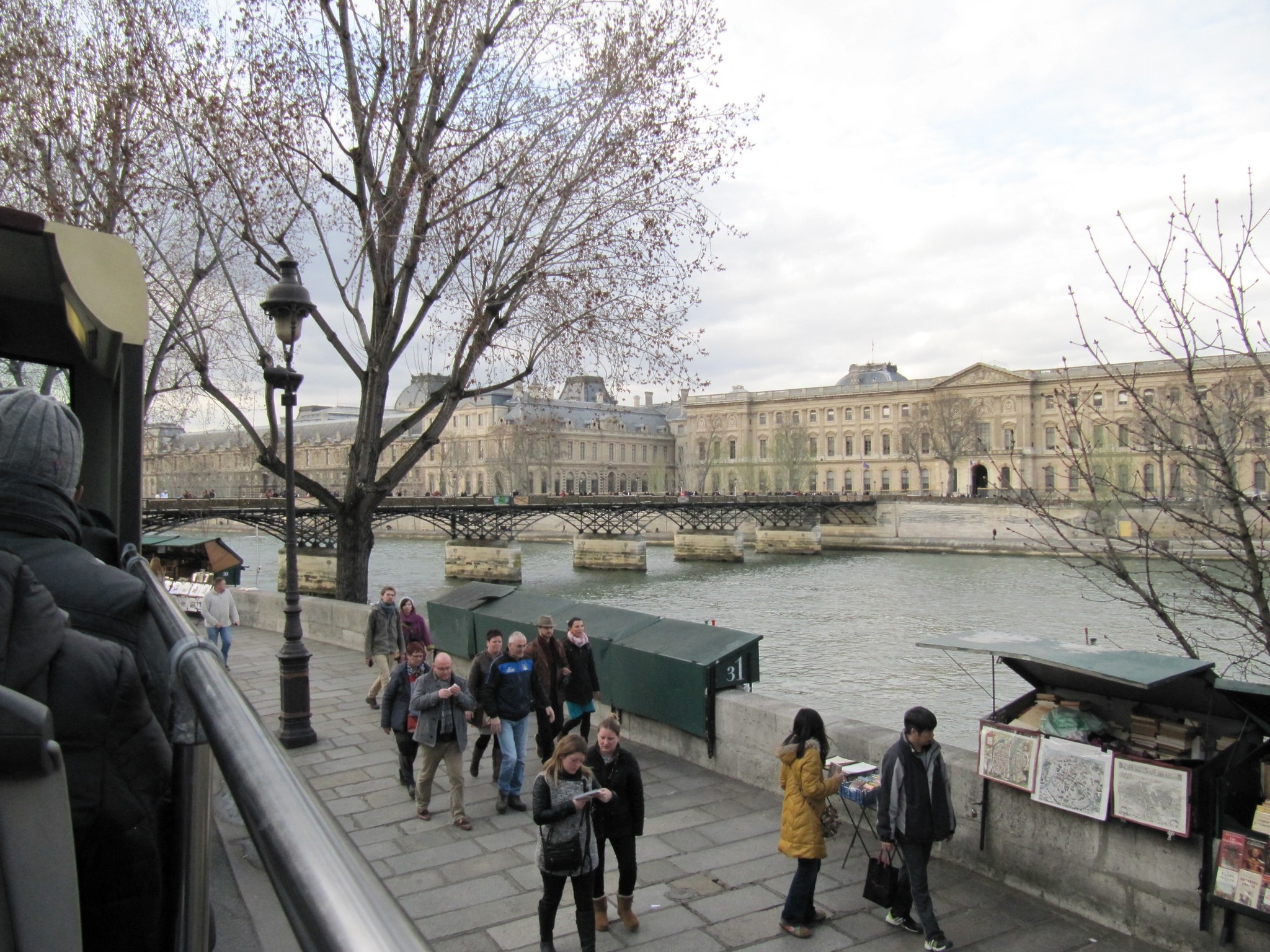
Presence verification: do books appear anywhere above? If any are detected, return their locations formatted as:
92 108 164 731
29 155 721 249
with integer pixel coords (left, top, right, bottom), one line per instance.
1029 690 1270 916
823 755 881 792
575 788 604 801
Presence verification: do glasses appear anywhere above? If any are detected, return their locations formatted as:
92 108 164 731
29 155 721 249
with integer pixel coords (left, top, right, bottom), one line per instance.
409 654 423 659
384 593 395 598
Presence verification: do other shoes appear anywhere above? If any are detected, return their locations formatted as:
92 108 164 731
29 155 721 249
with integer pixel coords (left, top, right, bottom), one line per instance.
804 907 827 921
457 818 472 830
365 697 380 710
407 784 416 797
417 808 430 821
535 734 544 757
495 791 507 813
778 918 811 939
507 794 527 812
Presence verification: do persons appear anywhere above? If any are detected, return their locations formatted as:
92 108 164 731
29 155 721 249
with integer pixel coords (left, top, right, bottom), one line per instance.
776 707 845 938
149 557 166 592
530 734 618 951
579 713 644 933
0 391 190 951
200 577 240 672
364 586 600 830
875 707 956 952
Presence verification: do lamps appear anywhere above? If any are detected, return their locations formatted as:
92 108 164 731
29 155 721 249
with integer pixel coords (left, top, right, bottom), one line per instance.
60 282 97 361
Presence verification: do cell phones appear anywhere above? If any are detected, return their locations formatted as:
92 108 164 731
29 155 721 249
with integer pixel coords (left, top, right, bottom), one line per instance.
574 790 602 801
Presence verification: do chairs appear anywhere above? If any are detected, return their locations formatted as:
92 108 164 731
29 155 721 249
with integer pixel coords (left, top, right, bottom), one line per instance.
0 684 82 952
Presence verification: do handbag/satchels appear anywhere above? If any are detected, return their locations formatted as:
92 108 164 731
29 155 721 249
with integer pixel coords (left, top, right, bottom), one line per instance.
541 834 583 871
862 847 900 908
466 702 485 726
820 806 841 838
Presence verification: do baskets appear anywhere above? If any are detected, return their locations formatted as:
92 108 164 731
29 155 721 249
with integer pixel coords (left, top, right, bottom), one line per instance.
843 777 881 805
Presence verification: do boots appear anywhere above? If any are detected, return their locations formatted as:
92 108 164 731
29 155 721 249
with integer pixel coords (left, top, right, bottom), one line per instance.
537 900 559 952
616 893 639 927
470 746 486 777
575 909 595 952
493 747 503 779
593 894 609 932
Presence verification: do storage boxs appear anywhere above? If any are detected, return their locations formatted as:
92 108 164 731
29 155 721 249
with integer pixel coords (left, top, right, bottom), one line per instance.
840 781 878 805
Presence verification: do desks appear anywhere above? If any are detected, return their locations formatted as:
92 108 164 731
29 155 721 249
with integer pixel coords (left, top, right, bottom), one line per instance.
824 755 878 807
837 792 903 869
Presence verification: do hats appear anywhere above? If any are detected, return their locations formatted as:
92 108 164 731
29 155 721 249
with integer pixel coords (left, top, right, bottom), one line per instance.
0 388 84 500
398 596 415 608
536 615 557 628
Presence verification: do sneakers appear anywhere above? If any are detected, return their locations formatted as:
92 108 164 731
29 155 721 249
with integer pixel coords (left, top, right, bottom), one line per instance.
885 911 924 933
925 935 954 951
